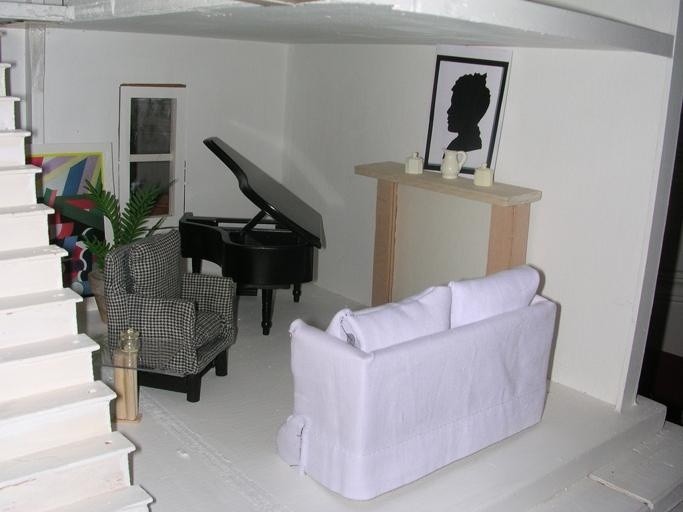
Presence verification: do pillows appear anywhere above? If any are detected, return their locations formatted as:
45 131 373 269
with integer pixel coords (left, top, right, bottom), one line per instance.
341 285 451 353
325 306 351 343
450 264 541 330
129 228 180 301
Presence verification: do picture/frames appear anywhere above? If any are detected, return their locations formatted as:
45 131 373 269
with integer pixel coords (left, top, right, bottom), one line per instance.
423 44 513 175
26 142 115 311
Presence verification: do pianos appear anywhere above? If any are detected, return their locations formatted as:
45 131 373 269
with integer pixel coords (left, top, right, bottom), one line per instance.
179 137 324 335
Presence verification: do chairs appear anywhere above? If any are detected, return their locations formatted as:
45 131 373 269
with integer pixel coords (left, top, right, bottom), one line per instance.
103 228 237 402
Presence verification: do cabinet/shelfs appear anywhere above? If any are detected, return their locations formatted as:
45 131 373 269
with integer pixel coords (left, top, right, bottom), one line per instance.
354 162 541 307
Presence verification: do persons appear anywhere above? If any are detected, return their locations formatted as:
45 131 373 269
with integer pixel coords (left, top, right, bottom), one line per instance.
443 72 489 158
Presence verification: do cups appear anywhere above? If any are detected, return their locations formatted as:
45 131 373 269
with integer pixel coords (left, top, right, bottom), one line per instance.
440 150 466 179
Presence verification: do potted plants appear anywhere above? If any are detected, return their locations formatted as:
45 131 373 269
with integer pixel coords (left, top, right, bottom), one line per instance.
80 179 178 324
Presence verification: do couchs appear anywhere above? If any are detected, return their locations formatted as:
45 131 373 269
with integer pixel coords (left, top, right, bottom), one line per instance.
276 264 558 501
